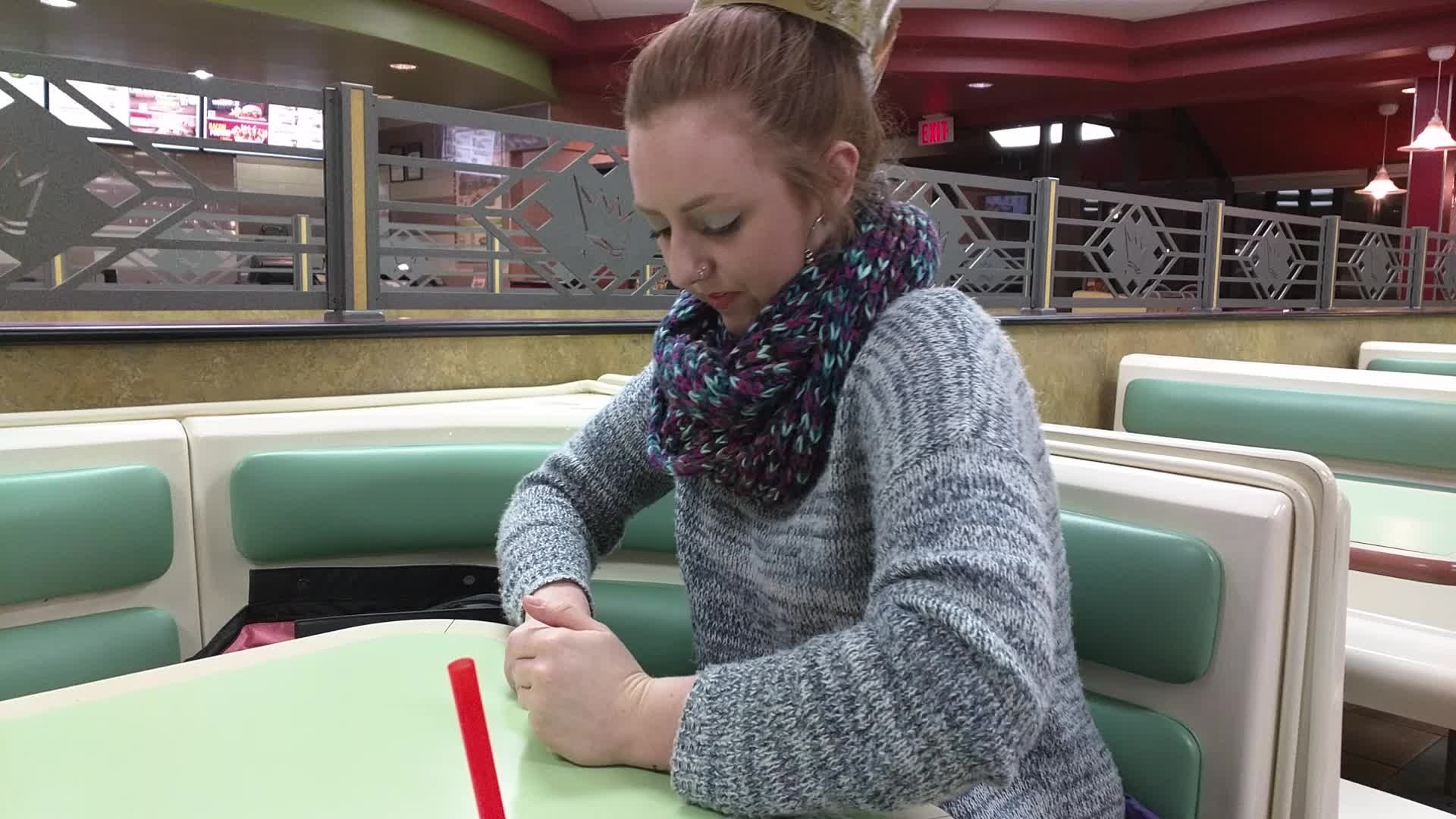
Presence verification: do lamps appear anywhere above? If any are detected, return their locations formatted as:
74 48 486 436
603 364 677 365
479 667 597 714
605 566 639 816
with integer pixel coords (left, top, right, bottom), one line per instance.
1396 44 1456 154
1352 102 1408 202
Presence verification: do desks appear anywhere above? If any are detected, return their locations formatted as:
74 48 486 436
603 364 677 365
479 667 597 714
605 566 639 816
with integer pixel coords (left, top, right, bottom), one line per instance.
0 612 957 819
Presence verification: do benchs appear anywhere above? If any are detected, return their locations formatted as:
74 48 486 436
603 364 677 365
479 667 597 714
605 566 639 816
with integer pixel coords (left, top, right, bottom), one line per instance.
0 335 1456 819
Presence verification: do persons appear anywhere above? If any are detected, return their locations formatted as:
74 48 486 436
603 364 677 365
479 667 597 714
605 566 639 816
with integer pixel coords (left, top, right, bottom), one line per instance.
495 0 1128 819
